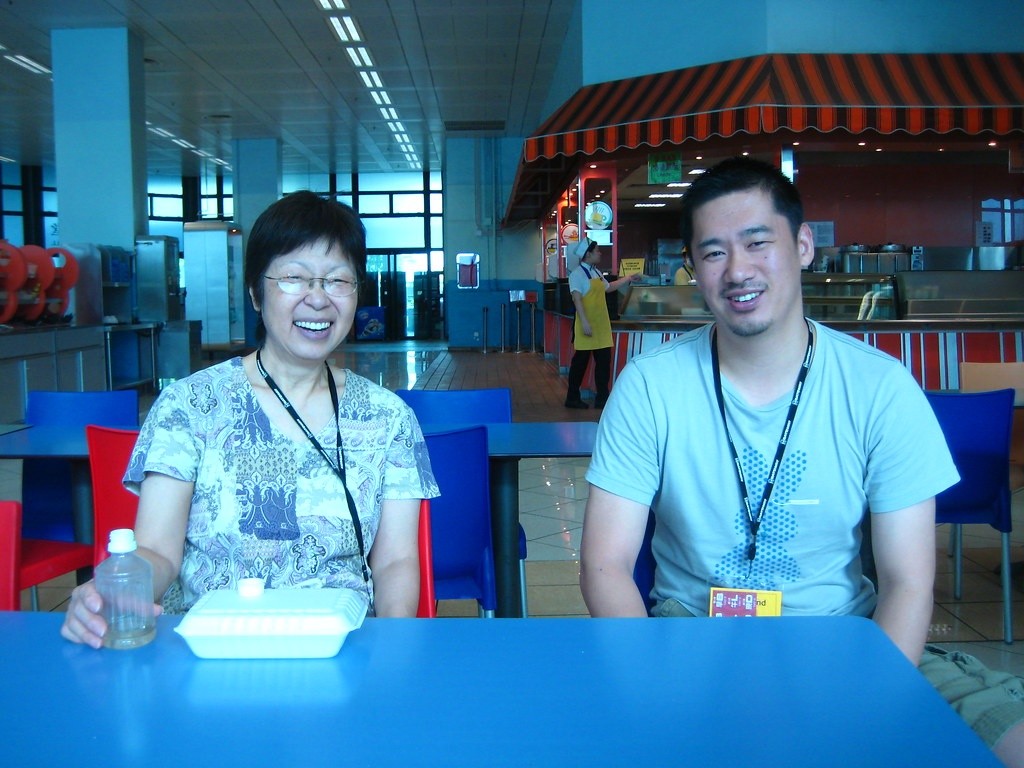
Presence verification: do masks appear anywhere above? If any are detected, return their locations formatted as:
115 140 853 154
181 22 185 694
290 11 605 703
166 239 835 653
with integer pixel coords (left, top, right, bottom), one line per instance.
686 258 693 268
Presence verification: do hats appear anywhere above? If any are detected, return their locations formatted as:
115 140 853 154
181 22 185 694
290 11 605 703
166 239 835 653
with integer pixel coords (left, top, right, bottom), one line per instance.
681 247 687 252
575 236 594 260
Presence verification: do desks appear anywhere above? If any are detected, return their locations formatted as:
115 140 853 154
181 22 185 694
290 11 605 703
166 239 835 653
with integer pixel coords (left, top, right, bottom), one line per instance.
0 421 609 619
0 612 1005 768
105 322 158 391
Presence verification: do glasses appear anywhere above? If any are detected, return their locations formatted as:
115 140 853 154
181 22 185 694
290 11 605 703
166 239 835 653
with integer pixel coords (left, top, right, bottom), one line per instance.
261 270 358 297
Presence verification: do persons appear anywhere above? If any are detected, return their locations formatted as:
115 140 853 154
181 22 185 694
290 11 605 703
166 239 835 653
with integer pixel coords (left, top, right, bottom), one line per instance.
564 237 639 408
580 158 1024 768
673 247 698 285
62 189 442 649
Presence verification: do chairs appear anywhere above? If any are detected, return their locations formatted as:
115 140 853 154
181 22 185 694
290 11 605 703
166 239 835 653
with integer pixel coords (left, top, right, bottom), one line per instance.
917 387 1016 644
946 360 1024 557
0 390 526 621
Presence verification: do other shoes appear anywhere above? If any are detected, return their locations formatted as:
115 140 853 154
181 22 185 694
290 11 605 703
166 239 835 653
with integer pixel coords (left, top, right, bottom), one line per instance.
565 400 589 409
594 400 606 408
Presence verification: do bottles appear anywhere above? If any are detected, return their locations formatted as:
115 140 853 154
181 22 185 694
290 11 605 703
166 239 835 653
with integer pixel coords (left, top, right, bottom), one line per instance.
93 529 157 649
